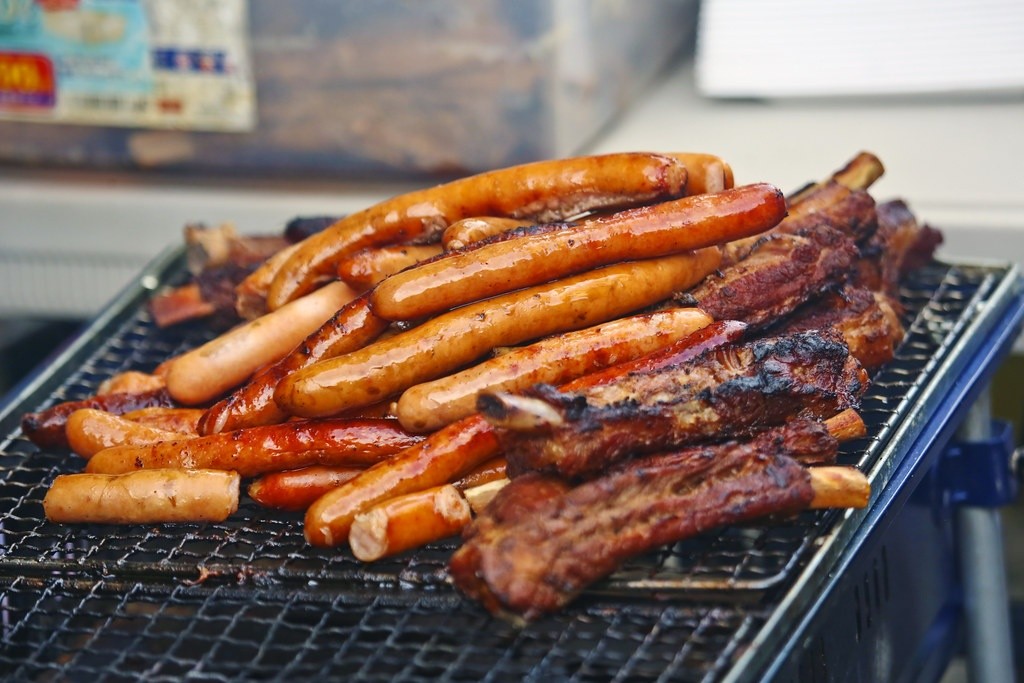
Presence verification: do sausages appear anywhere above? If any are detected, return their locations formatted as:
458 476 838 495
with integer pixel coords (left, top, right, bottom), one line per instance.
20 145 786 562
